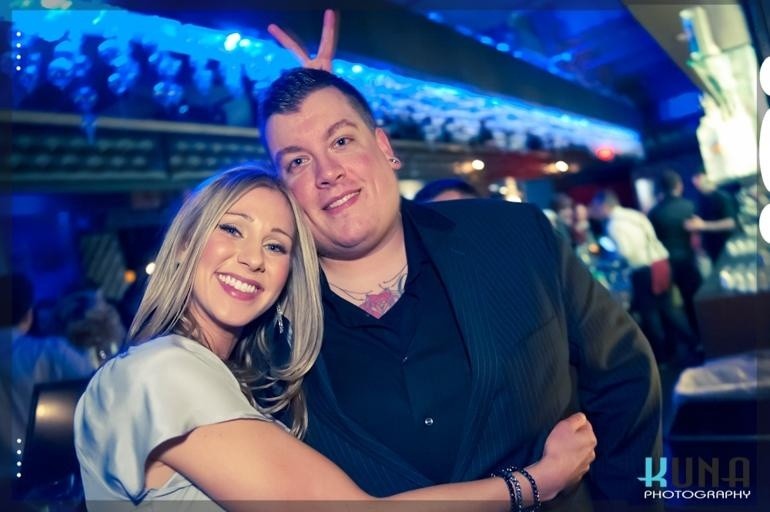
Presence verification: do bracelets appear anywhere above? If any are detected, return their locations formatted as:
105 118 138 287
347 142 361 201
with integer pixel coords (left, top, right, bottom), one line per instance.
491 464 541 512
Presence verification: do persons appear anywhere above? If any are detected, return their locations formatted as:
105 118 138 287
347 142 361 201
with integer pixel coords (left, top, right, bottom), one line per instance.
258 70 670 510
73 164 599 508
1 168 739 487
266 8 337 72
